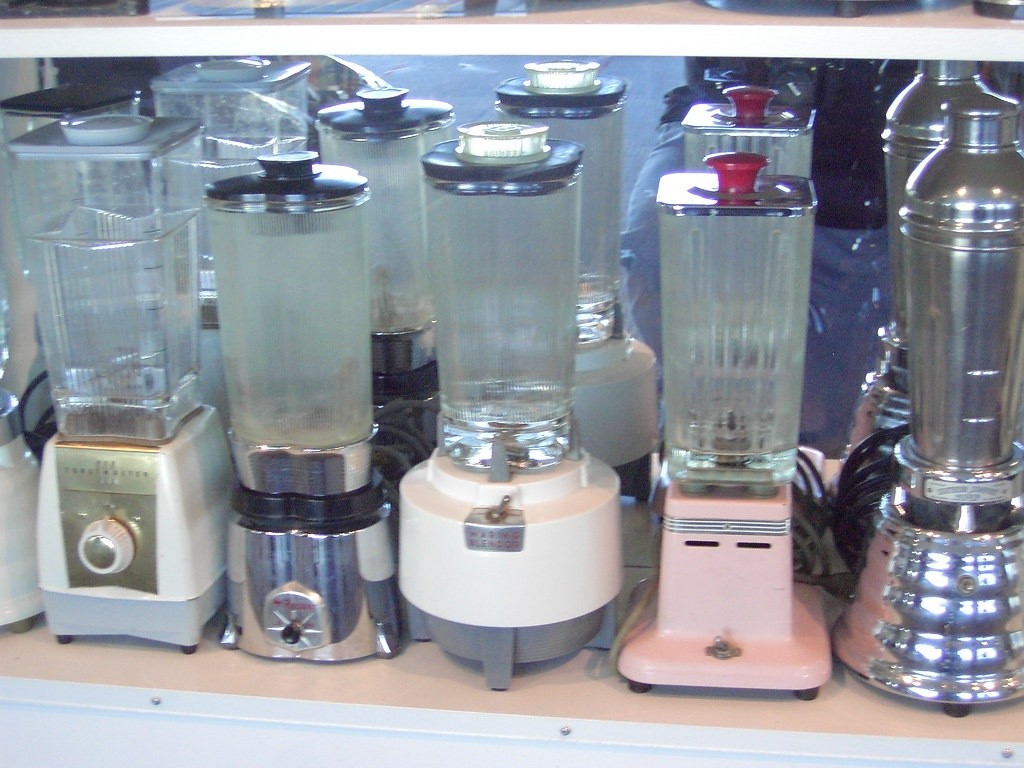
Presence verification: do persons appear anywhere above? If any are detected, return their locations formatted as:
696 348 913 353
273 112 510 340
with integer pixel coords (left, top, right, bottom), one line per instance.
618 0 1024 463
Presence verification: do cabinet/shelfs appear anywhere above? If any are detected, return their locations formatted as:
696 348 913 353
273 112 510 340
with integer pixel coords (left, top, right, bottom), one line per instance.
0 0 1024 768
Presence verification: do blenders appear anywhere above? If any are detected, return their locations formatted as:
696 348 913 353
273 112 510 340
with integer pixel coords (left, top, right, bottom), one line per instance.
0 56 1024 710
